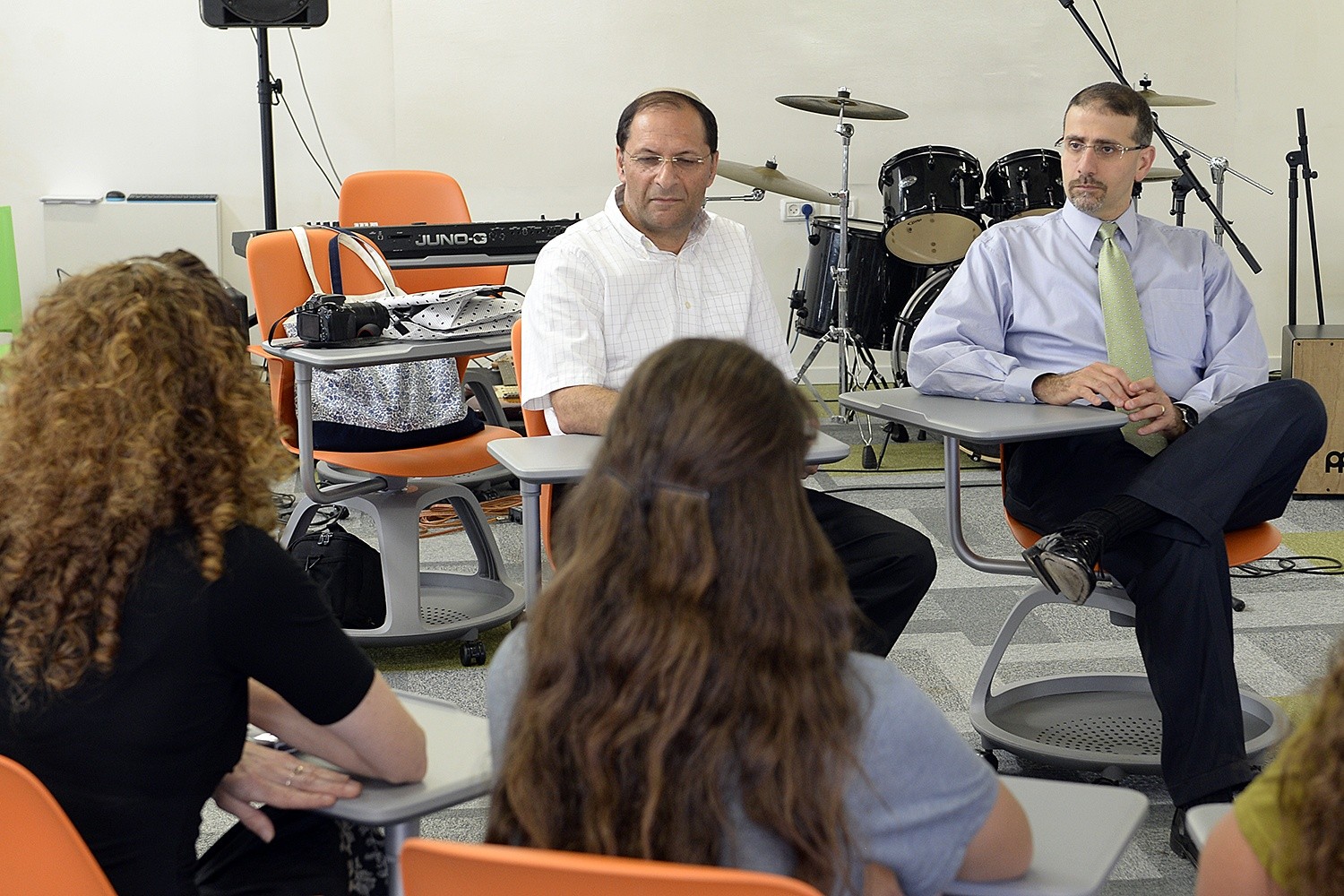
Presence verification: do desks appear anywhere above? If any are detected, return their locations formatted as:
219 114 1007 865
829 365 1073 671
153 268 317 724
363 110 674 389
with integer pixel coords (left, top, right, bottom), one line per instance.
244 688 493 896
838 386 1130 577
262 333 517 505
944 774 1149 895
486 423 850 621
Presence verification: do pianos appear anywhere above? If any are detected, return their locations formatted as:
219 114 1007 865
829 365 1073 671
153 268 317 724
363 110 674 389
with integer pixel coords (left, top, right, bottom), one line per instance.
230 211 582 270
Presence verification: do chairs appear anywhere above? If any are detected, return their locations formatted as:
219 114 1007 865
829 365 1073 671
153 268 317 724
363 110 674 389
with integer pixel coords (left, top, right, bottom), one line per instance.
970 445 1282 786
511 318 558 576
1 754 116 896
338 170 510 501
399 835 824 896
246 229 524 667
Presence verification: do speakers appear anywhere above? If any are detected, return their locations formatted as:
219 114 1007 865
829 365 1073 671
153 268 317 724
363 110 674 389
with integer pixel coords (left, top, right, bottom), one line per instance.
199 0 329 30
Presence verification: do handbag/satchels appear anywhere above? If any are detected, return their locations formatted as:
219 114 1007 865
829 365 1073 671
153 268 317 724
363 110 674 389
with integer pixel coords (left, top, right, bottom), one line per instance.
284 524 387 630
284 287 485 452
367 275 525 341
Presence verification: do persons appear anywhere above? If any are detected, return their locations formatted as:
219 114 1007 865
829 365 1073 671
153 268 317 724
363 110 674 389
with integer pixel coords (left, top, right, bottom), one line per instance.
909 81 1327 878
523 91 940 663
1195 633 1344 896
1 263 426 896
477 337 1030 896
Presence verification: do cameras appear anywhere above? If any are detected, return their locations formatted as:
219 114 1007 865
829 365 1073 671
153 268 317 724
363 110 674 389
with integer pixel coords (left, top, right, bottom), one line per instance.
296 293 391 344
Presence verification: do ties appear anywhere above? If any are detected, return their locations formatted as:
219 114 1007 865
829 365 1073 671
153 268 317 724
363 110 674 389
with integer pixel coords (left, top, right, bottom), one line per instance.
1098 219 1170 457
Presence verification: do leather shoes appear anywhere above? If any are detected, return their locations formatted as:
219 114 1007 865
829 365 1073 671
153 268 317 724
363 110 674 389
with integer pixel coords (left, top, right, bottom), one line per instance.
1020 524 1102 605
1169 785 1246 871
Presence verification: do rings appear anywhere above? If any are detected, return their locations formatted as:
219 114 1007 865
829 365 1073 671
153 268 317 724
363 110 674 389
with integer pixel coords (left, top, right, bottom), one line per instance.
296 764 305 775
286 774 296 788
1158 405 1168 417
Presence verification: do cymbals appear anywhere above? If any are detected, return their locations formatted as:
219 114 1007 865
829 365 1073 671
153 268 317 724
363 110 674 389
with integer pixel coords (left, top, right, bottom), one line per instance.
714 156 840 205
775 85 909 120
1139 167 1184 182
1131 72 1217 107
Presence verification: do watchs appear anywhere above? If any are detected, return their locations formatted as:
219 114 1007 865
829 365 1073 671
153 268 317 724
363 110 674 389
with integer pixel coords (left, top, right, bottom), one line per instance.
1174 403 1199 433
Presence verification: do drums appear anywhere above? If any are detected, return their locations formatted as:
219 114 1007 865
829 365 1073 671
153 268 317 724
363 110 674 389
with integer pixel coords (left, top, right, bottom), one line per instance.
878 144 987 268
891 265 1001 465
785 216 918 353
983 149 1067 222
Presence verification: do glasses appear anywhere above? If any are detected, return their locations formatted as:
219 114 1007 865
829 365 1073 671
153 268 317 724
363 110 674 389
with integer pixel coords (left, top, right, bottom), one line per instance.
622 147 715 168
1053 138 1147 162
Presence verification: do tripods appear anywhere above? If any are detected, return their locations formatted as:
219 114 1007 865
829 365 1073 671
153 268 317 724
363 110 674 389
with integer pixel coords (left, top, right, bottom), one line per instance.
792 125 908 470
